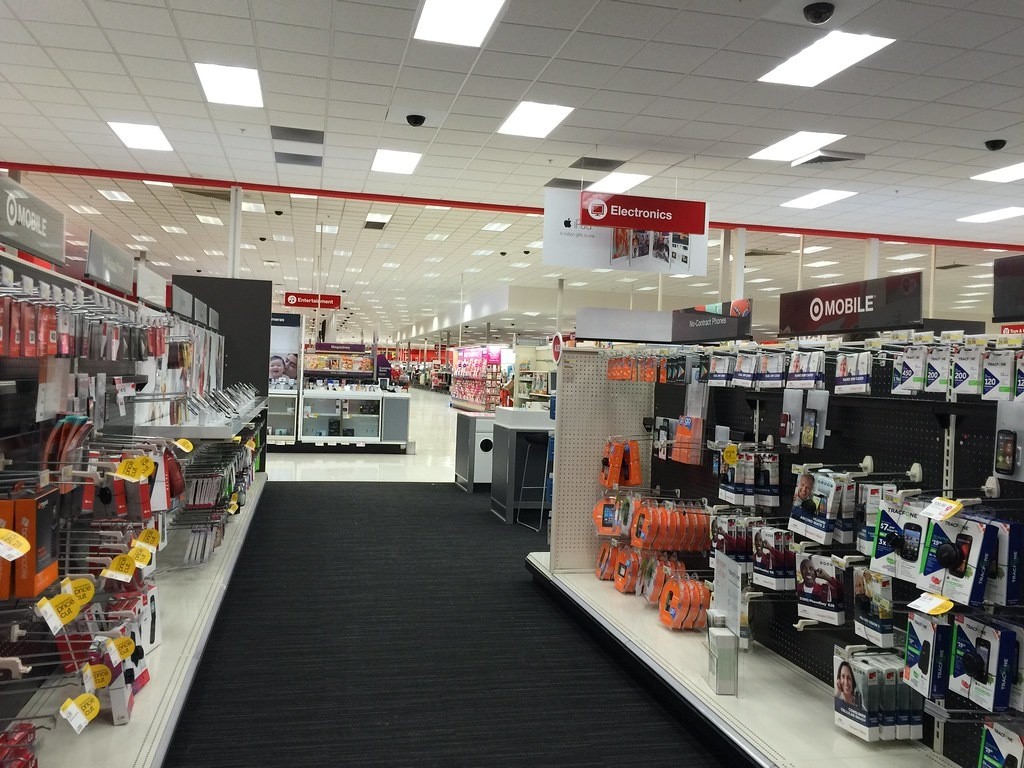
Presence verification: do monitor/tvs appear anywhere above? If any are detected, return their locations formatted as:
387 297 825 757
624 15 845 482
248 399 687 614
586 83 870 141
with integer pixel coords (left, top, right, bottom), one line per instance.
322 320 326 342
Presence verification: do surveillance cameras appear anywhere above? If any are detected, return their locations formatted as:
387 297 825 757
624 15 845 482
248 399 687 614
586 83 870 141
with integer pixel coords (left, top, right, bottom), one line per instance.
986 140 1008 151
310 290 353 337
275 211 283 215
803 2 834 25
524 251 530 254
260 238 266 241
407 115 425 126
197 270 201 272
500 252 506 256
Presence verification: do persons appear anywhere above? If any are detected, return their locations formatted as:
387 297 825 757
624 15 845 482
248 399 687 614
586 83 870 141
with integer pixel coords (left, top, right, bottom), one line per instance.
796 559 839 604
413 366 419 387
674 233 688 245
634 234 649 257
753 532 776 570
655 233 665 248
500 376 514 407
855 571 867 595
710 520 734 556
720 461 731 484
836 661 867 712
270 353 297 384
794 474 816 515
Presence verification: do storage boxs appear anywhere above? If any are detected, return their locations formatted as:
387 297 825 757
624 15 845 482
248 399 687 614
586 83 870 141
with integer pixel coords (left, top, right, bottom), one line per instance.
706 349 1024 403
592 415 711 631
0 487 59 601
56 584 162 725
0 296 165 363
712 450 1024 768
605 354 690 384
74 449 170 522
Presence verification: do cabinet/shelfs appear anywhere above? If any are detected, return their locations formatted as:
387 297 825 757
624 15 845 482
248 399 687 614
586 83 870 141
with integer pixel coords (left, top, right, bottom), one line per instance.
303 330 378 381
266 395 298 441
302 392 410 446
514 344 550 408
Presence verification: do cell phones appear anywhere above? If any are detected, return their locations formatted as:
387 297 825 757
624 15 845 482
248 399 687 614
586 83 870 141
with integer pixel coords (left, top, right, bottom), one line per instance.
974 637 991 684
609 228 691 271
901 523 922 561
949 534 973 578
995 429 1017 476
